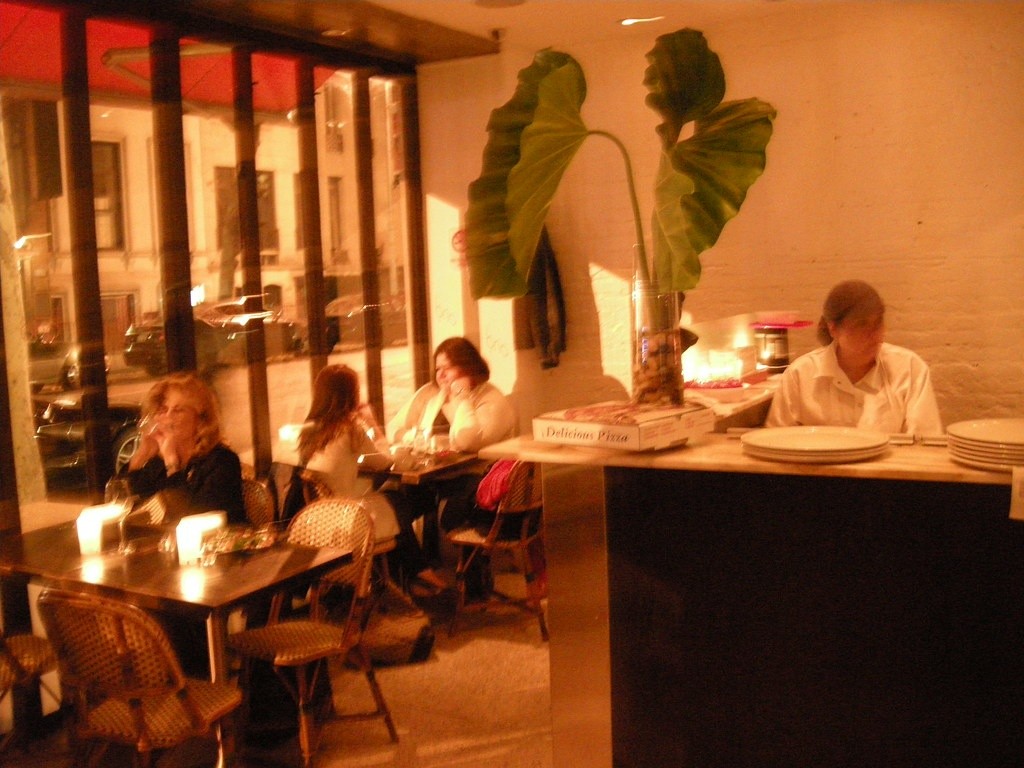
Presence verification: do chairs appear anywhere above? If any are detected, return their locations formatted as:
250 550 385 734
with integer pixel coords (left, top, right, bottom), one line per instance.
0 457 549 768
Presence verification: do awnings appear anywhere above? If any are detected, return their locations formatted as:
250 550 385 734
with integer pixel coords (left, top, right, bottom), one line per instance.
0 0 338 126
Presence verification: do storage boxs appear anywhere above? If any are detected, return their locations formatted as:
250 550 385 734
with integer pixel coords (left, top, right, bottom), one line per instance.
532 401 717 451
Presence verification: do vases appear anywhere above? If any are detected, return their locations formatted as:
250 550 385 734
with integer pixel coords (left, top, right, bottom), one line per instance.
630 272 683 407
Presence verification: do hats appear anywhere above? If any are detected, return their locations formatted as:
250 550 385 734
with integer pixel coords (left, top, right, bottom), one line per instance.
817 280 885 321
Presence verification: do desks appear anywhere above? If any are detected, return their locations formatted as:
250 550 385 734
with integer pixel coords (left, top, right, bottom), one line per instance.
1 518 353 768
243 450 481 559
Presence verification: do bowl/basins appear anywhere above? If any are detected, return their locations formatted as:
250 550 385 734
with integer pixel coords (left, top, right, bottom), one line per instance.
756 310 801 325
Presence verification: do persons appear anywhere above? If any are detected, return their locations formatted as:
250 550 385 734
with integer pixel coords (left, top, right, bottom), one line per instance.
127 372 244 681
762 279 944 435
385 337 517 604
296 365 450 599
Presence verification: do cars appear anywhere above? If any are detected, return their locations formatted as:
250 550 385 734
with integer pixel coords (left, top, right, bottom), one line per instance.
40 381 158 492
123 293 308 375
27 338 113 393
291 293 408 357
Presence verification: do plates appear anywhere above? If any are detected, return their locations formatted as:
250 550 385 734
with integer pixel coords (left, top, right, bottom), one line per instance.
747 321 813 329
946 419 1024 472
739 425 889 463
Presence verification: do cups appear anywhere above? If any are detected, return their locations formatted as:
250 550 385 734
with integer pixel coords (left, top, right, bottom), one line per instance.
137 408 165 436
431 435 450 463
